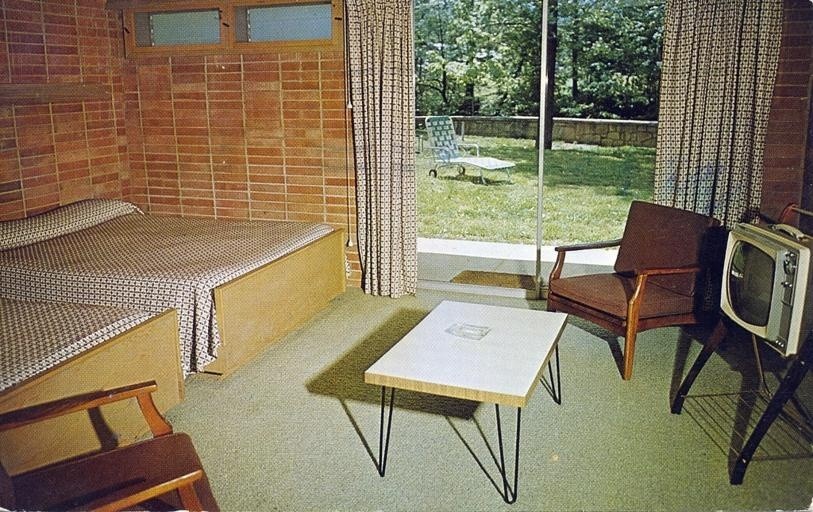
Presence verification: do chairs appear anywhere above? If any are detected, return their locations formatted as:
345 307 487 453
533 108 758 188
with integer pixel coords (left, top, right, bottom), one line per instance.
424 116 516 186
0 379 221 512
547 201 721 380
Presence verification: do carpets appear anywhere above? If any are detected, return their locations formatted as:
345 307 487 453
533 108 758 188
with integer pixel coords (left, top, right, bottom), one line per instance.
450 270 536 290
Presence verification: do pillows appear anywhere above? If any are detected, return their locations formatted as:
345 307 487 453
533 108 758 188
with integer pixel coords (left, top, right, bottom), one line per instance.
0 199 144 250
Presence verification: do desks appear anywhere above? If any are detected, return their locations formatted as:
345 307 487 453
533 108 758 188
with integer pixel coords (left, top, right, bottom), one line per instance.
364 299 568 504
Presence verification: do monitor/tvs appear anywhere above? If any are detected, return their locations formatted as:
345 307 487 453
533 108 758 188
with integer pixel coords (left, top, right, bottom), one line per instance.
720 222 813 361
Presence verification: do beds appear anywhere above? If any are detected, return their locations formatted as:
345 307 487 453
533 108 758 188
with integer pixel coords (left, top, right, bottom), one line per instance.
0 213 346 380
0 299 186 476
